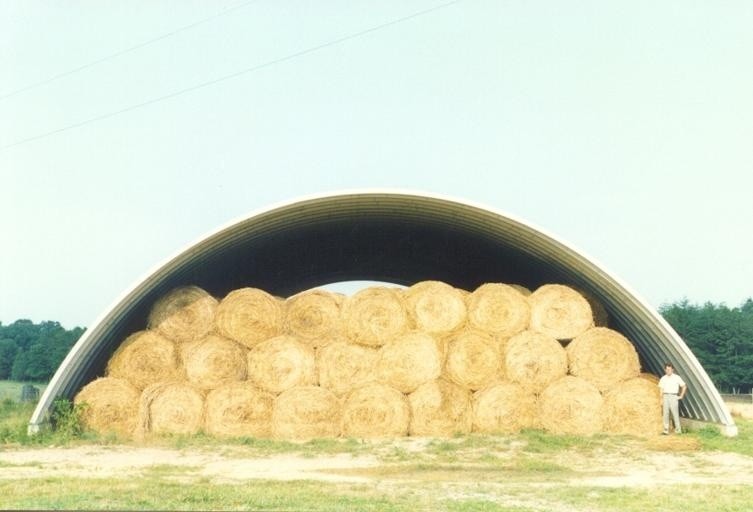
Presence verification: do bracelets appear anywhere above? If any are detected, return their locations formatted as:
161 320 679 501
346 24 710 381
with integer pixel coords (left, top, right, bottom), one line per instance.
659 395 662 398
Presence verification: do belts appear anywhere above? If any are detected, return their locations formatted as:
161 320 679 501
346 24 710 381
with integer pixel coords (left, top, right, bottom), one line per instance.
664 393 679 395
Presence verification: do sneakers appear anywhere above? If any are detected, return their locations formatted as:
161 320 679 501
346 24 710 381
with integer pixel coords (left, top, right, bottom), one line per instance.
675 428 681 432
663 429 669 434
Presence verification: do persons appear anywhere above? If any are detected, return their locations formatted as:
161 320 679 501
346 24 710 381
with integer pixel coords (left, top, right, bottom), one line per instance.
657 362 687 435
